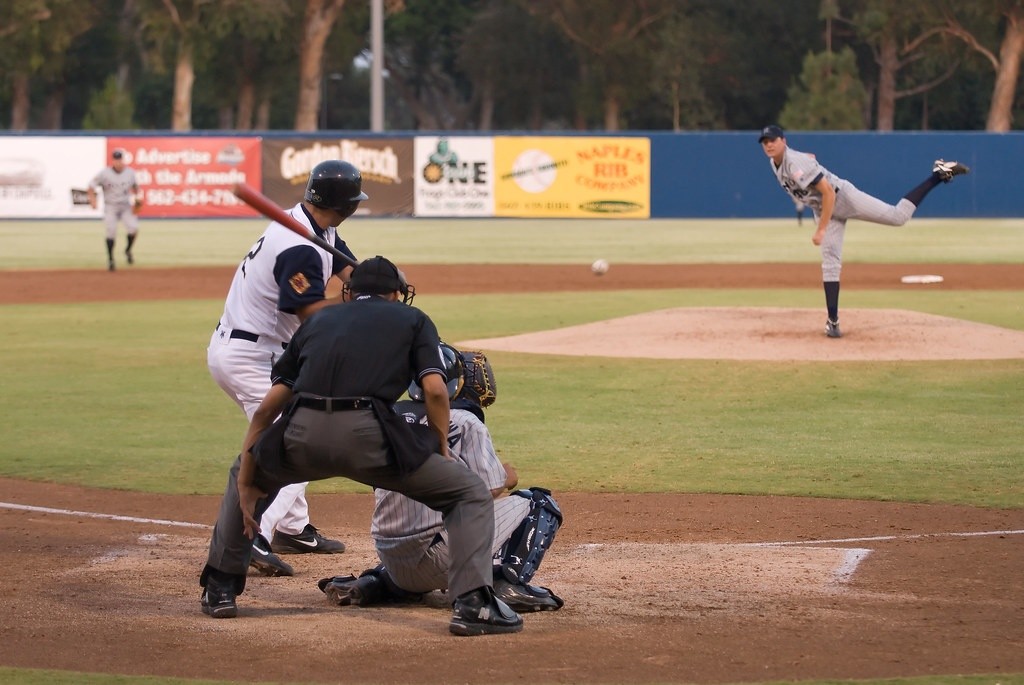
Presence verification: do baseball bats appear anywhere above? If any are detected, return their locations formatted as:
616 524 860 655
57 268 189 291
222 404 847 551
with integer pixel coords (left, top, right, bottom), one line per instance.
231 182 360 268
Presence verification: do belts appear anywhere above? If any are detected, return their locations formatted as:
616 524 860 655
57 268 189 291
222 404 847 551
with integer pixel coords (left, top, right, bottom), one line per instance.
835 187 838 193
298 396 374 411
429 533 442 548
215 322 289 350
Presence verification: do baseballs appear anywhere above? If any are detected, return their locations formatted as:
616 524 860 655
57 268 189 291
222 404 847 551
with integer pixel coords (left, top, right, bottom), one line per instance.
591 260 608 275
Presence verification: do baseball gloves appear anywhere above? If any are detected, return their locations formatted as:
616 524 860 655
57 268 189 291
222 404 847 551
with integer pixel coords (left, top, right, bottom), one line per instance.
457 350 497 410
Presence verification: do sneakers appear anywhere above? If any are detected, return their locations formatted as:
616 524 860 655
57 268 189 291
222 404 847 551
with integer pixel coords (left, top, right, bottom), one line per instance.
448 588 523 636
270 523 345 554
249 533 294 576
324 575 385 607
493 576 558 611
933 159 969 185
825 319 842 337
201 589 238 619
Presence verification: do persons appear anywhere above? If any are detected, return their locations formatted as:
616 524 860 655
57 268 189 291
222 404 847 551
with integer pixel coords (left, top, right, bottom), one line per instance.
198 255 524 636
87 151 144 271
207 160 370 572
318 342 565 613
759 125 970 337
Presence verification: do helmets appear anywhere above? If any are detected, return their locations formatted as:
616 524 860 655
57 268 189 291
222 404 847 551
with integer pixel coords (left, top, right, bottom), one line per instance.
408 347 457 401
304 160 369 218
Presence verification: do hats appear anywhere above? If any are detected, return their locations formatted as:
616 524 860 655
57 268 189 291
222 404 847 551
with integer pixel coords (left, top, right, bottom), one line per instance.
758 125 783 144
349 257 401 296
